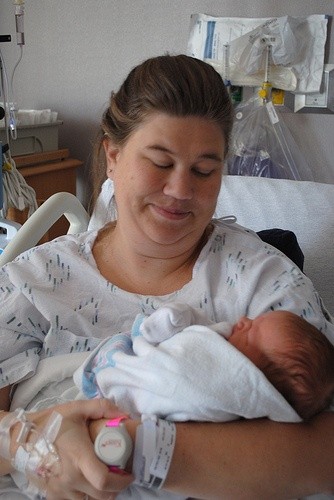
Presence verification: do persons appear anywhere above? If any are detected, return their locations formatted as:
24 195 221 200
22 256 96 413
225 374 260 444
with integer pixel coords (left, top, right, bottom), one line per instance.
10 300 334 500
0 54 334 500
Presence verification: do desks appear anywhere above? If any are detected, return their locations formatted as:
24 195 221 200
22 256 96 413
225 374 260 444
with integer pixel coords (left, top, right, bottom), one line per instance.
1 159 82 246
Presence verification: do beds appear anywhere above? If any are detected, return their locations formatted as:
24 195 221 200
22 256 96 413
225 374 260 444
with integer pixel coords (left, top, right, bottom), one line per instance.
0 174 334 500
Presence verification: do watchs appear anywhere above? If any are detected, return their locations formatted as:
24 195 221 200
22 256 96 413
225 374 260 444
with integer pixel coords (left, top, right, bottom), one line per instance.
94 416 132 476
